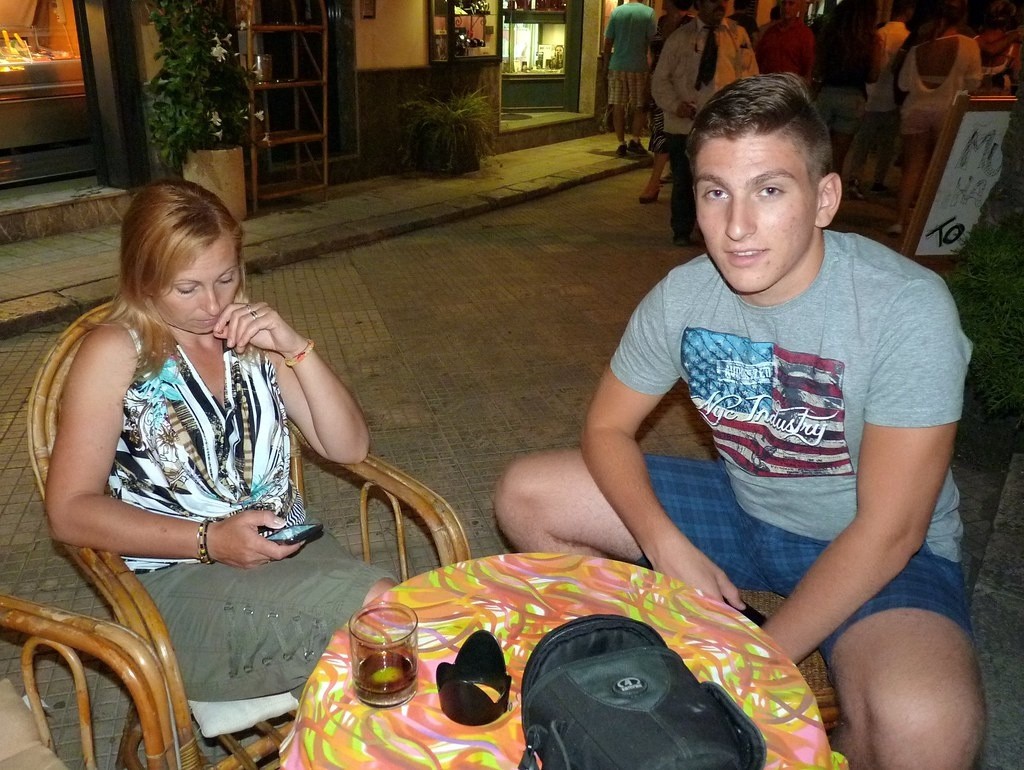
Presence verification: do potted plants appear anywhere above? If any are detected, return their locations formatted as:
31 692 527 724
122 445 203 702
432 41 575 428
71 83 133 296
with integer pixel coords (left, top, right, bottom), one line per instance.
403 85 498 173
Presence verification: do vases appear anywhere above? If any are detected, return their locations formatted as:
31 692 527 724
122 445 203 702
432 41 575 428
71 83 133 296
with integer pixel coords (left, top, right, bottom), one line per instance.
180 147 247 224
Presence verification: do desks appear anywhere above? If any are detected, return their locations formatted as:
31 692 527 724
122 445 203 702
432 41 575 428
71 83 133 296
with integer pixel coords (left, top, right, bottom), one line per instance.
279 551 849 770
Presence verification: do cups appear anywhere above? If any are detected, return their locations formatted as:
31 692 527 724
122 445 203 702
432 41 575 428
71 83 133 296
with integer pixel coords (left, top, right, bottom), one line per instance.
347 602 418 707
246 53 272 81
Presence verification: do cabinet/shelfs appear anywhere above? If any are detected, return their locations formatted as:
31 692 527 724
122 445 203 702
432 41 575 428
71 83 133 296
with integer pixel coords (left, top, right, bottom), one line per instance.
427 0 503 65
248 1 329 201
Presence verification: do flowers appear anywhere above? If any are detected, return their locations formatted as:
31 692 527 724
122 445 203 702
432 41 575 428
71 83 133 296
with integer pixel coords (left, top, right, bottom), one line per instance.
143 0 269 144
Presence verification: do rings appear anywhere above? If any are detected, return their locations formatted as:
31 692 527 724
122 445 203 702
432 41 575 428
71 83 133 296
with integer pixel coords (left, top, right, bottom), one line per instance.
246 304 252 312
251 311 258 320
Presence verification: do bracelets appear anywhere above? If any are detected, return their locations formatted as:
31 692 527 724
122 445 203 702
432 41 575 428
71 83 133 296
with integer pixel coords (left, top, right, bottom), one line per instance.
196 520 216 565
285 339 315 366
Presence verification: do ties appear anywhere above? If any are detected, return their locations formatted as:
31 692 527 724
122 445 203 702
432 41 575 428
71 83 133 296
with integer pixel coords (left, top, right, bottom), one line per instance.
694 26 718 91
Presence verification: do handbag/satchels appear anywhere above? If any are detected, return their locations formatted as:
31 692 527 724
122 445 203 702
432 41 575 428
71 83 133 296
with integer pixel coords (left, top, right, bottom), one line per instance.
518 616 767 770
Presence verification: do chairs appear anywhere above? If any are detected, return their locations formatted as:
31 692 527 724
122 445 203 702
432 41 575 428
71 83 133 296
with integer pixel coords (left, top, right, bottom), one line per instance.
30 302 471 770
1 596 179 770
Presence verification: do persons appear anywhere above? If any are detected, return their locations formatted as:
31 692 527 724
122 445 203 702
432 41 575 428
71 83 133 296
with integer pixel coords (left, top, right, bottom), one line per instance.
555 45 564 57
602 0 657 155
494 75 985 770
46 179 402 701
639 0 760 248
887 0 984 238
733 0 1024 200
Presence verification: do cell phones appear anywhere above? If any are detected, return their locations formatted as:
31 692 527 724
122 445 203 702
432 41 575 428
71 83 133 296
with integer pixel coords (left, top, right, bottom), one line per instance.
266 523 324 545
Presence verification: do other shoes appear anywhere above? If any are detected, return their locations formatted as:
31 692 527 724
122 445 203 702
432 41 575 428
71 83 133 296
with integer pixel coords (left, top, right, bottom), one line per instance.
886 223 902 236
638 186 659 204
616 144 627 155
628 139 649 156
661 172 676 184
893 153 904 167
847 177 864 199
673 234 690 247
871 176 890 195
